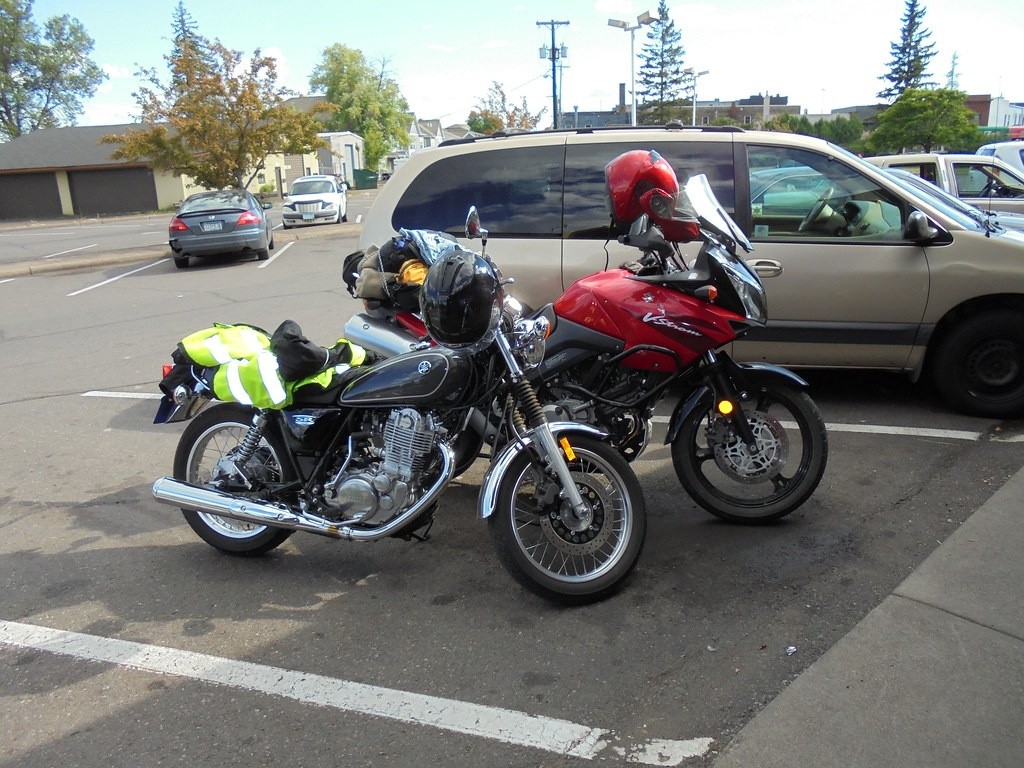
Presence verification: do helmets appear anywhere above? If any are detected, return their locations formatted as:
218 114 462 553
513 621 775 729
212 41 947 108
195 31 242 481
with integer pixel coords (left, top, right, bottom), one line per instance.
419 252 504 355
603 149 701 244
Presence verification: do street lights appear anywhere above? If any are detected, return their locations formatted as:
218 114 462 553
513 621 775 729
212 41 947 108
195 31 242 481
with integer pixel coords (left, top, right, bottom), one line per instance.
608 11 661 127
539 42 567 130
683 67 709 127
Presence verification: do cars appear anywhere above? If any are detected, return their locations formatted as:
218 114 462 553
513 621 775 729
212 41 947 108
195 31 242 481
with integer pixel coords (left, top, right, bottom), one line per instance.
282 175 348 228
168 189 275 269
965 125 1023 201
747 164 852 215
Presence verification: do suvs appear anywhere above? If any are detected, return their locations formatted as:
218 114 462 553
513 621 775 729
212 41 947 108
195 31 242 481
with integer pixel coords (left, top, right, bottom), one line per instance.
859 150 1024 225
359 116 1022 420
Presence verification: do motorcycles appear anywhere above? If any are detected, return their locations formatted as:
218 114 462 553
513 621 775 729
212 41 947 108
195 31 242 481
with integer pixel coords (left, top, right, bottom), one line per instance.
340 150 829 529
151 206 647 606
